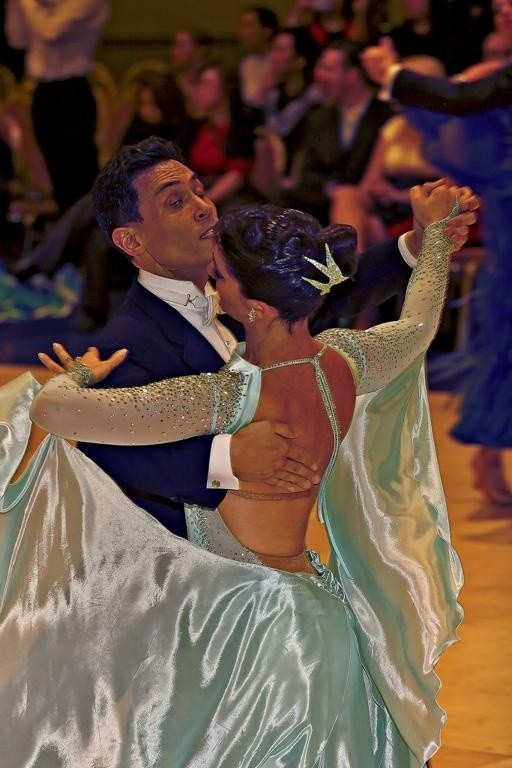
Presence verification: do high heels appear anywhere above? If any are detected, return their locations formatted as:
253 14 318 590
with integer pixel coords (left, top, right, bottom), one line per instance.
465 452 511 509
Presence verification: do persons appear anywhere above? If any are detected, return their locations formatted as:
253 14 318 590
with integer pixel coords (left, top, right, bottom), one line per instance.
67 131 485 555
0 174 476 768
1 1 512 511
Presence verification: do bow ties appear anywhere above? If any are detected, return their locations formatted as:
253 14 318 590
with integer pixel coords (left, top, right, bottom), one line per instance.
137 278 224 326
